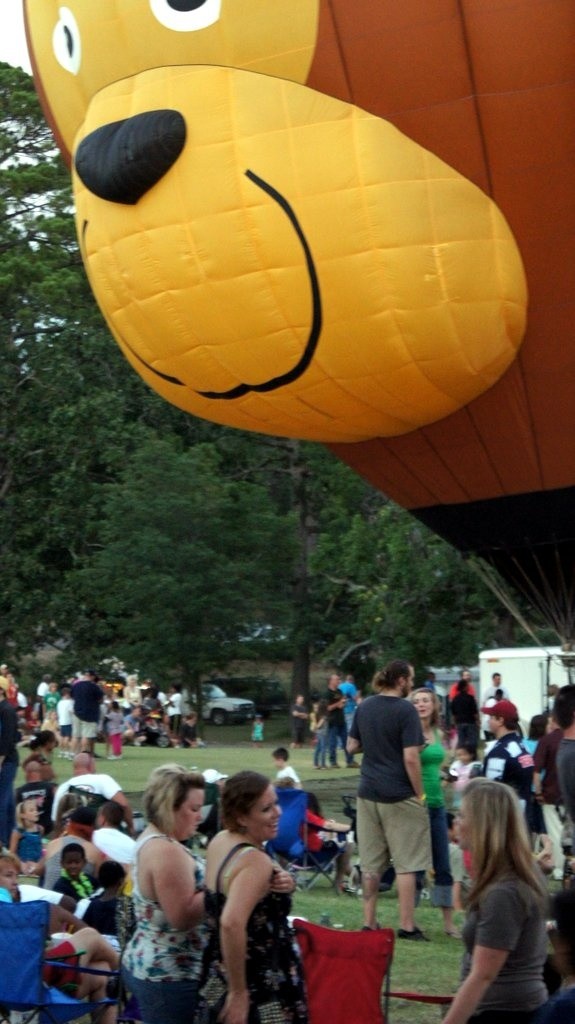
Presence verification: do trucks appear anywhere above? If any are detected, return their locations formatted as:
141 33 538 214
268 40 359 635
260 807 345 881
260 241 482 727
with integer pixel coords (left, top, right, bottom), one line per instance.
477 646 575 742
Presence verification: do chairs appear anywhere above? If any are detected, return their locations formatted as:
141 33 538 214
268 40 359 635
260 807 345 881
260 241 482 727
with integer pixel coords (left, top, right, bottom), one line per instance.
0 900 123 1024
270 788 355 896
292 919 456 1024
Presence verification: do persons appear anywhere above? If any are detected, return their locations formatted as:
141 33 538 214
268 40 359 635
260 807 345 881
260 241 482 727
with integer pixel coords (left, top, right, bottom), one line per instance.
120 763 207 1024
442 775 549 1024
0 659 575 1024
345 659 433 942
190 770 310 1024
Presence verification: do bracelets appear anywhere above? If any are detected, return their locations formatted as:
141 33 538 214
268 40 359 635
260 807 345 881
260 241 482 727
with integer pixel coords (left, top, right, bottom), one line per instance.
421 792 426 800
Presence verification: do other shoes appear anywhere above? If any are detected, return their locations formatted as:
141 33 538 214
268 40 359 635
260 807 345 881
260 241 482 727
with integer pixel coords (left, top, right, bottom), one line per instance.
314 765 329 770
398 926 430 942
330 764 340 768
69 752 76 760
346 761 361 768
445 927 463 938
361 922 380 931
107 753 122 760
58 751 69 758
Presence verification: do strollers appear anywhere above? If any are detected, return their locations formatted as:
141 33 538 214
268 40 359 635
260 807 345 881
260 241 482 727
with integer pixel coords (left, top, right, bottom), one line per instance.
130 703 171 748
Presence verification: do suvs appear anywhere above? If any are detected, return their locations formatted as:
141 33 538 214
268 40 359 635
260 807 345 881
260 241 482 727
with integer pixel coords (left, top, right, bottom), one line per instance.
200 675 290 719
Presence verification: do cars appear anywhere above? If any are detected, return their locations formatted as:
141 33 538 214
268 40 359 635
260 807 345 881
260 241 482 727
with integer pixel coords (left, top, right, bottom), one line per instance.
220 626 327 658
198 685 258 726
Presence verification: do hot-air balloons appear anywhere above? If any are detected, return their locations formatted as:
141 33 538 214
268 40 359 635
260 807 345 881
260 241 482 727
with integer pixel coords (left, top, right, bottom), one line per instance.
23 0 575 694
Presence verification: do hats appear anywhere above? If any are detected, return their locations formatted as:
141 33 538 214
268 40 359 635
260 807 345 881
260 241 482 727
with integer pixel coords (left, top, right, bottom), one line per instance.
480 700 519 724
61 806 96 826
202 768 229 784
